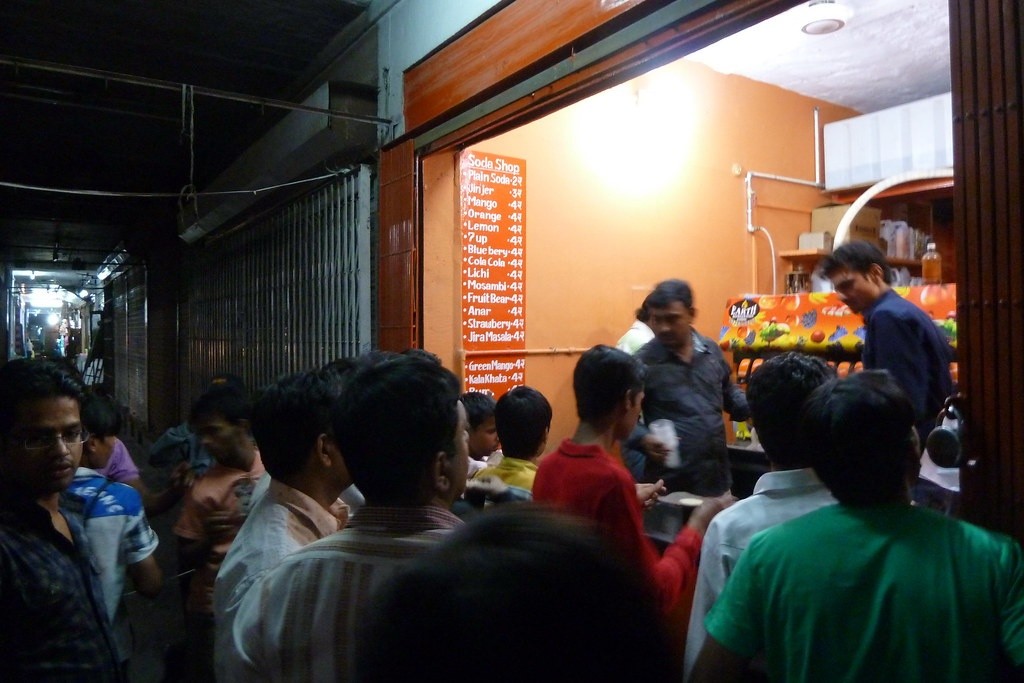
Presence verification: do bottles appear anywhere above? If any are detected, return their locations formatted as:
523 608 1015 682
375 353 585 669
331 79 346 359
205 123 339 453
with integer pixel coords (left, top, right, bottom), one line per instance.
921 243 941 285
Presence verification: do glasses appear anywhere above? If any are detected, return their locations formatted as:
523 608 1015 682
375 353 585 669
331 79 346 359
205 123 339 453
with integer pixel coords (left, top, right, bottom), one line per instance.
0 423 90 449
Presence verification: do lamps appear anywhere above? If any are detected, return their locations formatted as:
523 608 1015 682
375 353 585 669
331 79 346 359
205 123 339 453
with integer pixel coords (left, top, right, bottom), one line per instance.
801 0 855 37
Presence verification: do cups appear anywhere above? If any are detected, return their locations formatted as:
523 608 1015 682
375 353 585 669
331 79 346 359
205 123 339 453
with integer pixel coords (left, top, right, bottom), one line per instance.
647 418 679 469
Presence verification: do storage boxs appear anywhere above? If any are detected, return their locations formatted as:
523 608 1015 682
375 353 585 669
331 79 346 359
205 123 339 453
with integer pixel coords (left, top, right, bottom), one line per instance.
799 231 833 249
811 201 883 245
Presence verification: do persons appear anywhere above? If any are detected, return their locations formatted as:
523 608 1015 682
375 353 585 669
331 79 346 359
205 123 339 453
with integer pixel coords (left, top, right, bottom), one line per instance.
1 282 1024 683
817 238 953 513
